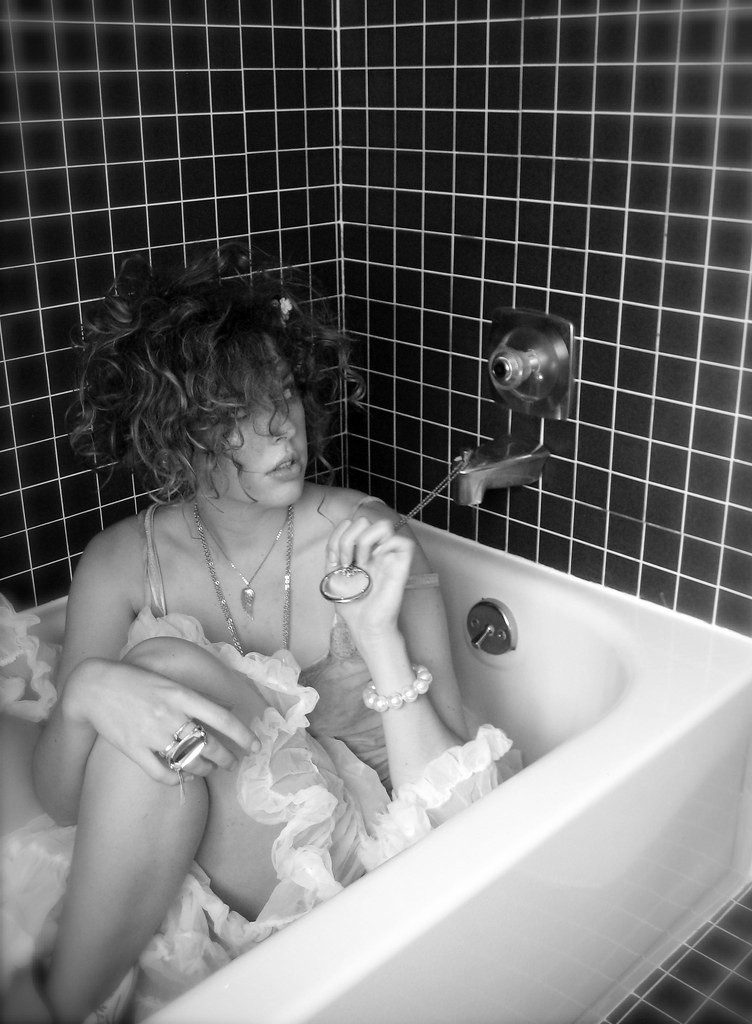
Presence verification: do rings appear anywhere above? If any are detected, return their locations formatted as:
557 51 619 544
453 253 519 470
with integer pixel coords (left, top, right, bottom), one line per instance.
159 720 202 761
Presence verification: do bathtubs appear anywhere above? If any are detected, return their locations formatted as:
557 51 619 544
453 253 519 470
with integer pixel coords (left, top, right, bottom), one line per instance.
0 515 752 1023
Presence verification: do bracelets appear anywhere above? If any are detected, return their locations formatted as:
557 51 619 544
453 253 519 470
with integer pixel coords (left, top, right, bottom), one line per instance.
362 661 431 712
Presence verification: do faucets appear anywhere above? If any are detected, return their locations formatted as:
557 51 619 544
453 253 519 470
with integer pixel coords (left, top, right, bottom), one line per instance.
455 434 550 506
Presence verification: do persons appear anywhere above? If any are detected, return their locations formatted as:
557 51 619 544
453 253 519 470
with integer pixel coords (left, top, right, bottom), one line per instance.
0 242 523 1024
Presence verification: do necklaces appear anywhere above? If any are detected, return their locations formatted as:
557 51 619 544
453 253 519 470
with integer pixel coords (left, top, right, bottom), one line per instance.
194 499 294 655
